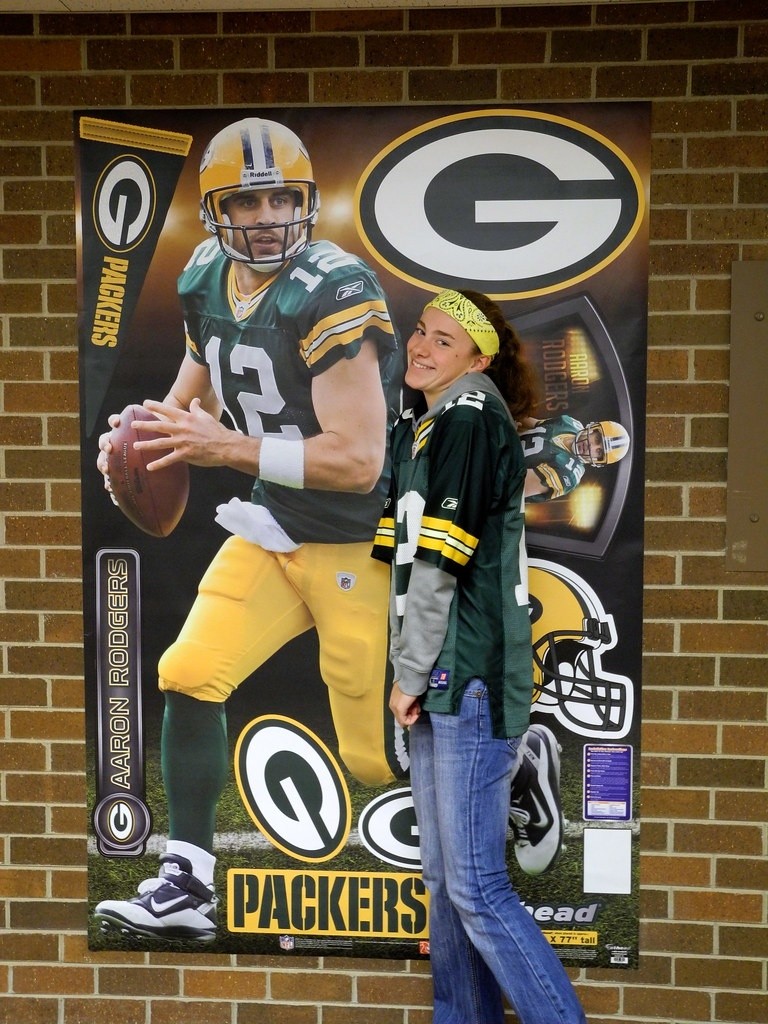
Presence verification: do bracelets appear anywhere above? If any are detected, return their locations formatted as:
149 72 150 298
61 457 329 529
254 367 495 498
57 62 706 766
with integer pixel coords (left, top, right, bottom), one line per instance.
258 436 306 489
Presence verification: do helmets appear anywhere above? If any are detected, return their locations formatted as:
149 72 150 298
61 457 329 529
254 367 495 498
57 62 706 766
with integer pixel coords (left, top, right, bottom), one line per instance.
199 117 315 245
597 420 630 468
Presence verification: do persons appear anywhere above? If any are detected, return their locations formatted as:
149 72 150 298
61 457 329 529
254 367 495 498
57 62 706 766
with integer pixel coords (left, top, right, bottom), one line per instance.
518 417 631 502
93 115 562 944
370 289 587 1024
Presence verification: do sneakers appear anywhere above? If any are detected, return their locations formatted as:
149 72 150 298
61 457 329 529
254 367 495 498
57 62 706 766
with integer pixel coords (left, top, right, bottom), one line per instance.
507 724 564 875
93 853 216 944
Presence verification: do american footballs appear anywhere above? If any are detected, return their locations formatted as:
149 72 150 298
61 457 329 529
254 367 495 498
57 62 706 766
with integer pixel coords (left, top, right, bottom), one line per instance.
107 403 190 538
